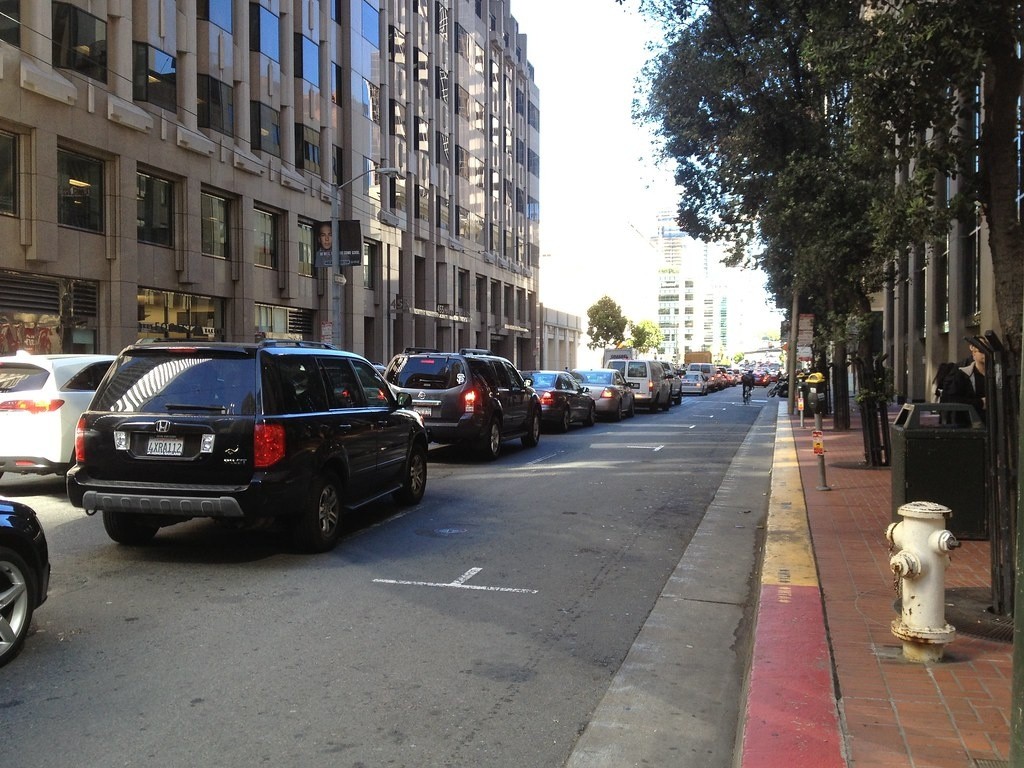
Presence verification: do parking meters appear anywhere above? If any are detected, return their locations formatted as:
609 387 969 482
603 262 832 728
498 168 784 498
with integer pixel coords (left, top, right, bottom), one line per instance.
796 373 809 428
806 372 832 492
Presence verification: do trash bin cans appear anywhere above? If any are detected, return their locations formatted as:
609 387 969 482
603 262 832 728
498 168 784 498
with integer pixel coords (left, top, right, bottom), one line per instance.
795 372 829 418
889 401 993 542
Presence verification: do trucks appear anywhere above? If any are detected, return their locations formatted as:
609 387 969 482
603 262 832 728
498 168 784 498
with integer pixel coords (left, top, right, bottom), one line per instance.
604 347 641 369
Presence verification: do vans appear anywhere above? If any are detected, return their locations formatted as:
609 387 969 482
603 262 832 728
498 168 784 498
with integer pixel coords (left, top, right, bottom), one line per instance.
605 360 674 413
687 363 718 393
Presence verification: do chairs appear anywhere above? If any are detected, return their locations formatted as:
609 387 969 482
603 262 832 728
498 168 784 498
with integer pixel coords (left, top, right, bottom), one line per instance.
285 373 341 413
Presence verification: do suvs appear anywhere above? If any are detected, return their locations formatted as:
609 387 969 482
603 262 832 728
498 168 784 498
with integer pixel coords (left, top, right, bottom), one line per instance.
66 333 428 552
383 348 542 464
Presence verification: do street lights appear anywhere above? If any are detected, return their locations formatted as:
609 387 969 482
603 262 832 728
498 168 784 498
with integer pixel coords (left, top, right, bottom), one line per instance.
332 168 397 348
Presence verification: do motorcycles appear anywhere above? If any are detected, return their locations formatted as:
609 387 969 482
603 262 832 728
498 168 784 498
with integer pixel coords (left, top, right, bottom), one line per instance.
767 378 788 398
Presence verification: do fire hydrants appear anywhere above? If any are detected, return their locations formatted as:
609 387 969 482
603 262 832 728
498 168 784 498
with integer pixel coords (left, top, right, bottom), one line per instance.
886 501 961 664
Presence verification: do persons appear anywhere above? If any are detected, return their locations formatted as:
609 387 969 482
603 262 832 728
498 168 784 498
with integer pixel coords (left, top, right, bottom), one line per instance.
944 336 987 426
743 370 754 402
315 222 332 267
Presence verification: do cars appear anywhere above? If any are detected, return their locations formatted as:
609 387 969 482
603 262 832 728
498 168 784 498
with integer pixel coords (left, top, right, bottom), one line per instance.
0 350 118 479
571 368 635 421
661 361 686 407
715 367 780 389
0 499 51 668
519 370 596 433
680 371 709 396
